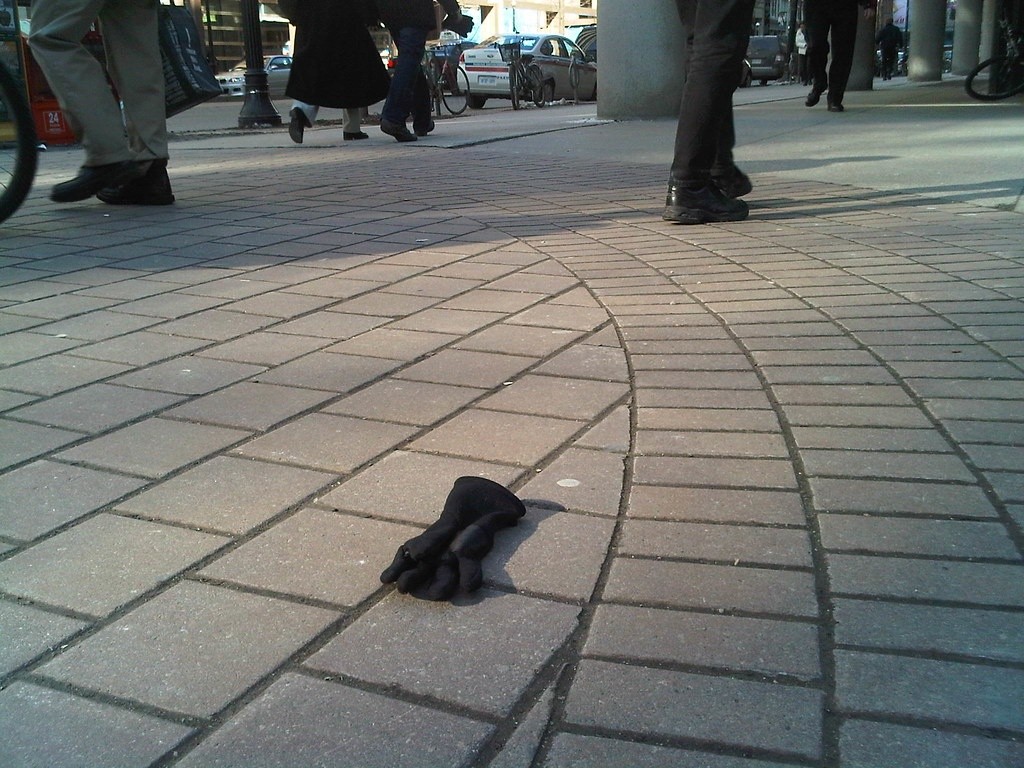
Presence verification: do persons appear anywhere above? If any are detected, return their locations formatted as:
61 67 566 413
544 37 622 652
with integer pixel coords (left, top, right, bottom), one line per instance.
876 18 903 81
795 21 812 86
27 0 174 205
277 0 391 143
374 0 463 142
802 0 878 111
662 0 756 223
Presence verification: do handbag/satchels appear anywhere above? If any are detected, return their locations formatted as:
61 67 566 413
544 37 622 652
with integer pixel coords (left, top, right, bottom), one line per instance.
152 4 222 118
424 3 441 41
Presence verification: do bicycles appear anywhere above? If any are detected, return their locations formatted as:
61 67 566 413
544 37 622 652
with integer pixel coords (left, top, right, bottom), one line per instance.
875 43 902 82
489 37 547 109
964 6 1024 99
421 42 472 114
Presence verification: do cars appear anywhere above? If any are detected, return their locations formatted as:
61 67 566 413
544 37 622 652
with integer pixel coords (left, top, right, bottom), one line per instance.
212 55 293 97
428 40 478 69
457 32 597 108
744 32 785 85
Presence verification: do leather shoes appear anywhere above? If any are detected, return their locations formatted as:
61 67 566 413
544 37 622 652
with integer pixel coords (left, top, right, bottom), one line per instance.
662 178 749 224
711 166 752 199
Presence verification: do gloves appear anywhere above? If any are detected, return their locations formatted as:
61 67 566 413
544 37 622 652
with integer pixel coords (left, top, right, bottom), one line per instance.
381 475 527 601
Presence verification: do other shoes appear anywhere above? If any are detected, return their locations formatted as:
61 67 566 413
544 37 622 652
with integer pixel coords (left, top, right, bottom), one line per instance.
381 118 417 141
415 119 434 136
343 131 368 140
828 102 843 112
289 108 307 143
49 158 135 202
97 161 175 204
804 79 828 106
